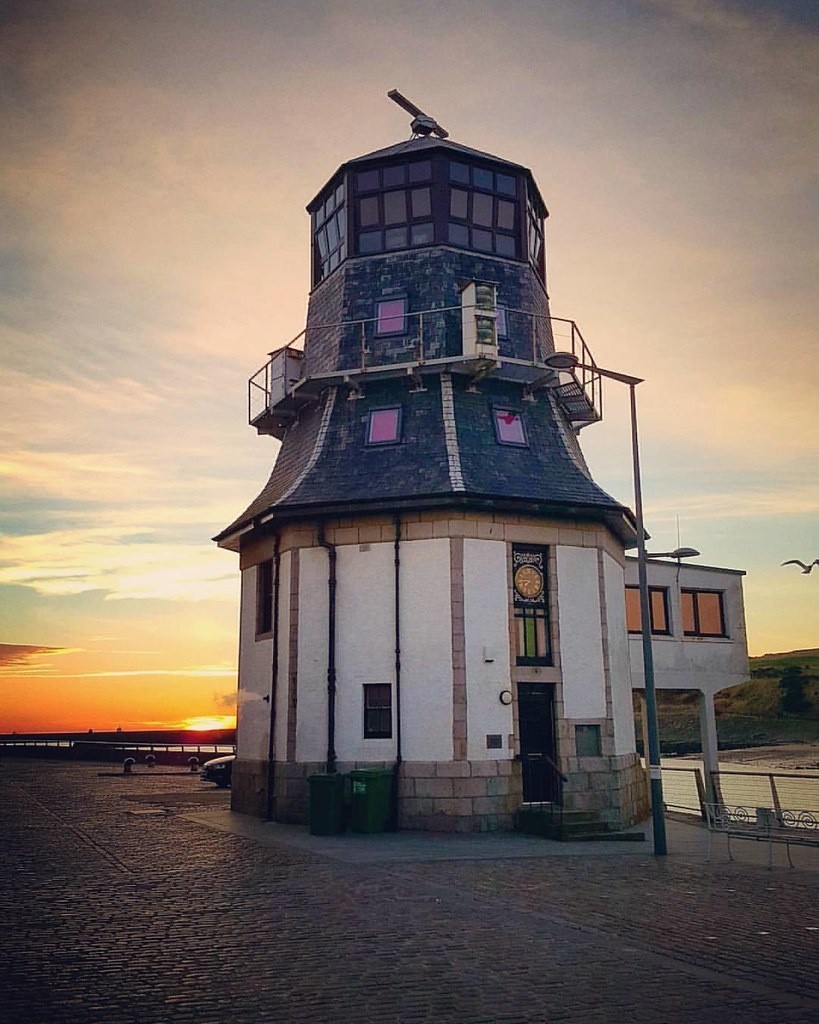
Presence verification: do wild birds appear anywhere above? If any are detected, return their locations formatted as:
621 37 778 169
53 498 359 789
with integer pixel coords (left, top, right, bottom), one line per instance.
780 558 819 574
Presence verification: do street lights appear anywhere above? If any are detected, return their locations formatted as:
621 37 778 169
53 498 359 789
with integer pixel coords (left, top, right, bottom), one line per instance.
539 348 700 855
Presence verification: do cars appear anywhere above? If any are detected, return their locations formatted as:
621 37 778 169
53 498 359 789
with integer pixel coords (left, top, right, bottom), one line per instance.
199 755 236 788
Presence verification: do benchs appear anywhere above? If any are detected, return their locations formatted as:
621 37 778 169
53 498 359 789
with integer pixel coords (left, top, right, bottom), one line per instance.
702 801 819 869
